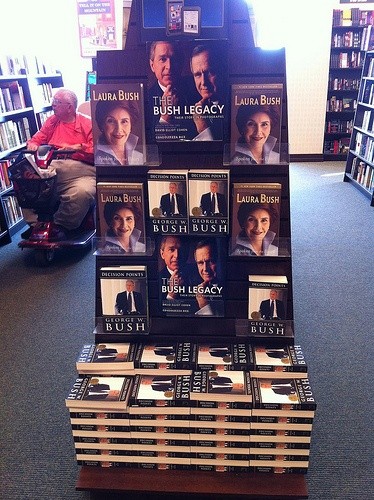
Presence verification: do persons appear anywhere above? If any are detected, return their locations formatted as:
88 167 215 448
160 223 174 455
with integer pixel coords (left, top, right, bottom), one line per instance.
84 40 302 402
21 88 96 241
15 163 40 180
95 193 146 253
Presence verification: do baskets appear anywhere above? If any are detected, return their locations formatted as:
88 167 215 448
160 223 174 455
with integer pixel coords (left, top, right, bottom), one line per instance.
9 171 56 208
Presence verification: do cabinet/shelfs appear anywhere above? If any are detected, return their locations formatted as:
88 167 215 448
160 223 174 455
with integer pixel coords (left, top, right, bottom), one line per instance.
0 70 66 248
323 25 374 161
342 49 374 206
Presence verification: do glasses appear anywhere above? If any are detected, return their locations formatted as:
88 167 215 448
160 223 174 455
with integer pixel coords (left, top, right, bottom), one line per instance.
52 98 68 104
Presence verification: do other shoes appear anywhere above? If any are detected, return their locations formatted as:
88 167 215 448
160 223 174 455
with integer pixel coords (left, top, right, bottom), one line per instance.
20 227 32 240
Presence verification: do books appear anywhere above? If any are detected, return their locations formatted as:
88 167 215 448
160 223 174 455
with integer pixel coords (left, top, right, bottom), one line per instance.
64 81 317 474
326 8 374 197
0 51 65 233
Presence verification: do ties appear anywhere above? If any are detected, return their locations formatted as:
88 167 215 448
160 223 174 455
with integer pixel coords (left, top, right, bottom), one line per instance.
153 383 171 384
271 386 291 387
127 292 132 312
171 194 175 214
156 348 173 350
268 351 283 352
269 300 274 318
212 383 230 386
212 193 215 212
211 350 228 351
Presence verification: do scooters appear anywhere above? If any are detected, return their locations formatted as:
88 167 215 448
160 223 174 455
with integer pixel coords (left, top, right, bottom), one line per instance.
8 145 96 268
